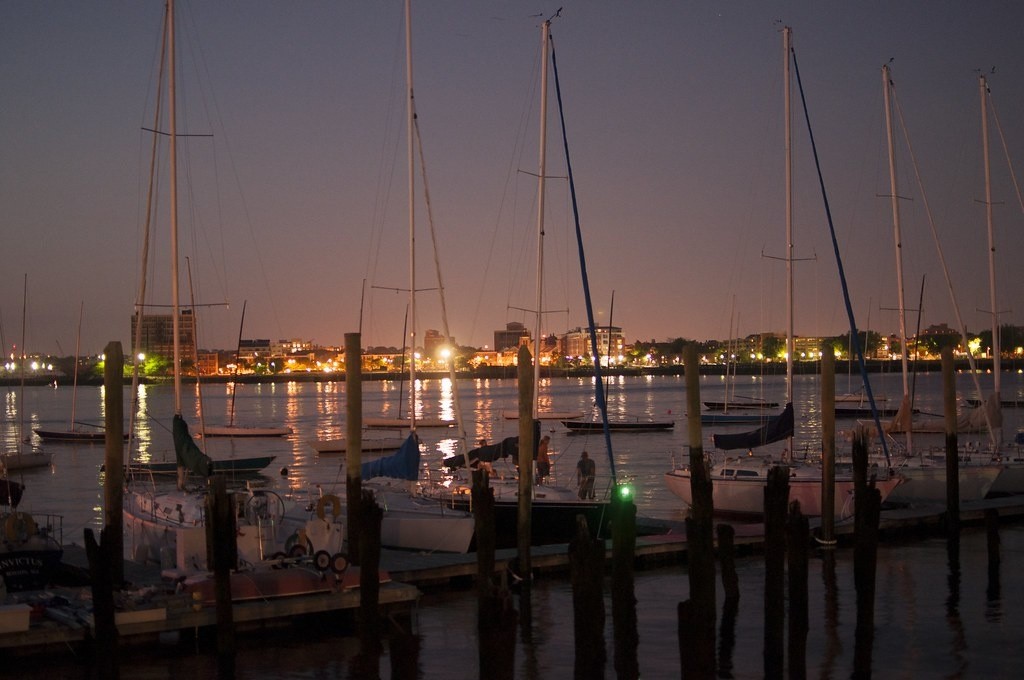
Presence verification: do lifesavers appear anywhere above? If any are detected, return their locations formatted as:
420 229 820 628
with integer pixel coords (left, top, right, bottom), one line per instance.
317 495 341 519
5 512 36 545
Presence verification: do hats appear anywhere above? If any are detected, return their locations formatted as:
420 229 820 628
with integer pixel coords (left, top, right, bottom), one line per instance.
581 451 588 457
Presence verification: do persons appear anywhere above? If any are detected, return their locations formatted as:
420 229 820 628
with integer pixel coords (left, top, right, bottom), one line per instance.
478 439 493 478
536 435 551 487
576 451 596 501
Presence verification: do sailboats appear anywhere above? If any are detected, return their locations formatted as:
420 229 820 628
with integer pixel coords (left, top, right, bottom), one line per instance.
31 299 136 443
0 270 55 472
99 1 1024 553
